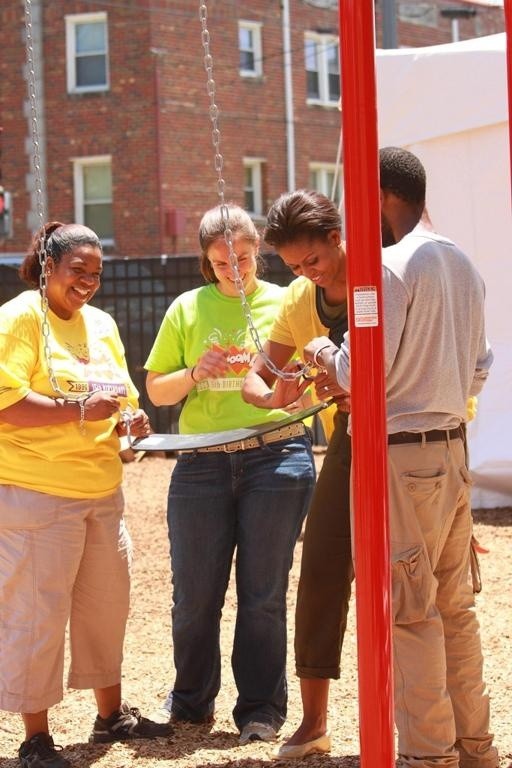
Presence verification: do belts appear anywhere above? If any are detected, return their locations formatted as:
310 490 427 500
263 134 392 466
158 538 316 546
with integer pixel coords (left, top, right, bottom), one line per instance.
387 425 465 445
177 419 308 456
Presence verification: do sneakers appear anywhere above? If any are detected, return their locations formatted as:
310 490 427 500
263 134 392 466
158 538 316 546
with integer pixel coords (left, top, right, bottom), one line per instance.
145 705 179 726
235 721 278 745
17 731 73 767
91 701 174 744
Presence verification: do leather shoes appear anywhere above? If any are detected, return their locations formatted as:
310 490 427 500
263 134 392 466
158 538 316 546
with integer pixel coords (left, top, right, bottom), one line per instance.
270 728 332 760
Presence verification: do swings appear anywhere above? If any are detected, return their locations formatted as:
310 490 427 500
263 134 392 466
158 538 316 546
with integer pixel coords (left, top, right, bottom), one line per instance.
23 0 349 452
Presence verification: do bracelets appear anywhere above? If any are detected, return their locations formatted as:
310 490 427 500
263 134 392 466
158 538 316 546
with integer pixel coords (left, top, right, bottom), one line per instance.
190 365 198 383
78 400 85 430
314 344 331 367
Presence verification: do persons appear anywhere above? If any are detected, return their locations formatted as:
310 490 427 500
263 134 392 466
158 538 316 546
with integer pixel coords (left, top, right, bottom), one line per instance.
0 220 173 767
303 146 500 768
143 204 317 746
242 189 346 761
